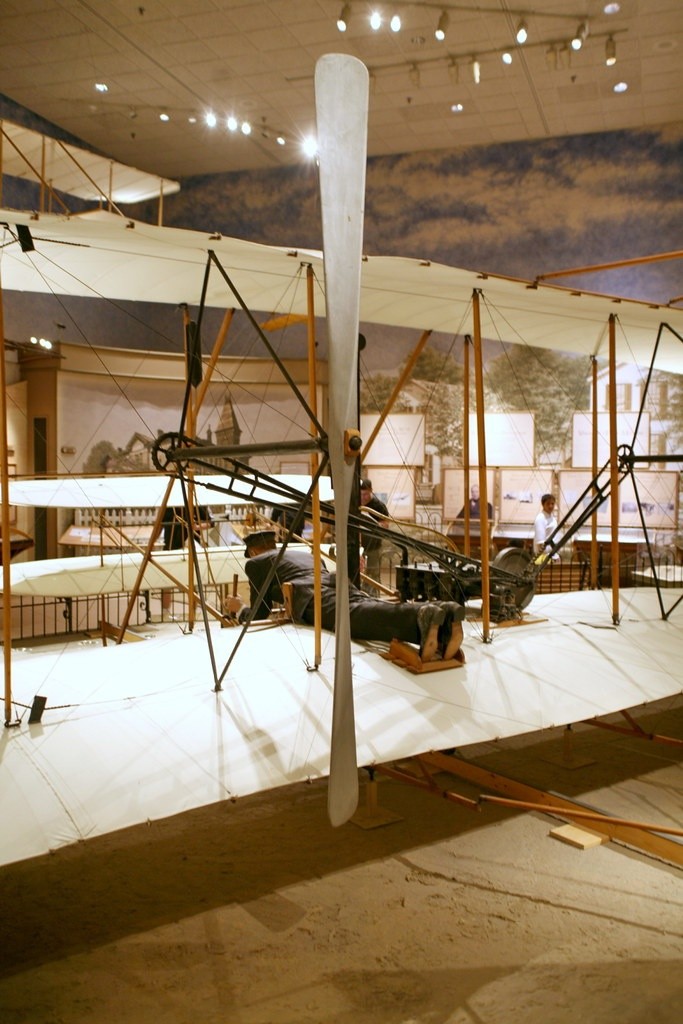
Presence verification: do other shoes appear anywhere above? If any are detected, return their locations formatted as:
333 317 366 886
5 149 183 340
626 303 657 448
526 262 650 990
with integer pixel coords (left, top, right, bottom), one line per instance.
417 604 447 662
442 601 466 660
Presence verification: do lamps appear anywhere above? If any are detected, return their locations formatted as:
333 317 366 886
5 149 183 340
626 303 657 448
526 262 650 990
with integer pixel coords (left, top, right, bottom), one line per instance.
0 224 35 252
57 98 287 145
338 0 617 90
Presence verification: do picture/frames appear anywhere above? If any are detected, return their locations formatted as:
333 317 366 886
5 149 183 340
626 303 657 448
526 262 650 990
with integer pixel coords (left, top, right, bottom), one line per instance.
440 464 679 531
360 465 417 523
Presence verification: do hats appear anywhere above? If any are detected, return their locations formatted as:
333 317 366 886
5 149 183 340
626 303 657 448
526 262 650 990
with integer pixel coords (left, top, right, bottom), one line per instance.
243 531 276 558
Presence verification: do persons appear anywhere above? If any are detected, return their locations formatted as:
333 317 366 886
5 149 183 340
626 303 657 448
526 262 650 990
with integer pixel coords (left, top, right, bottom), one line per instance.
266 505 306 543
532 494 564 564
457 484 492 519
361 479 389 599
160 507 214 550
226 531 465 661
